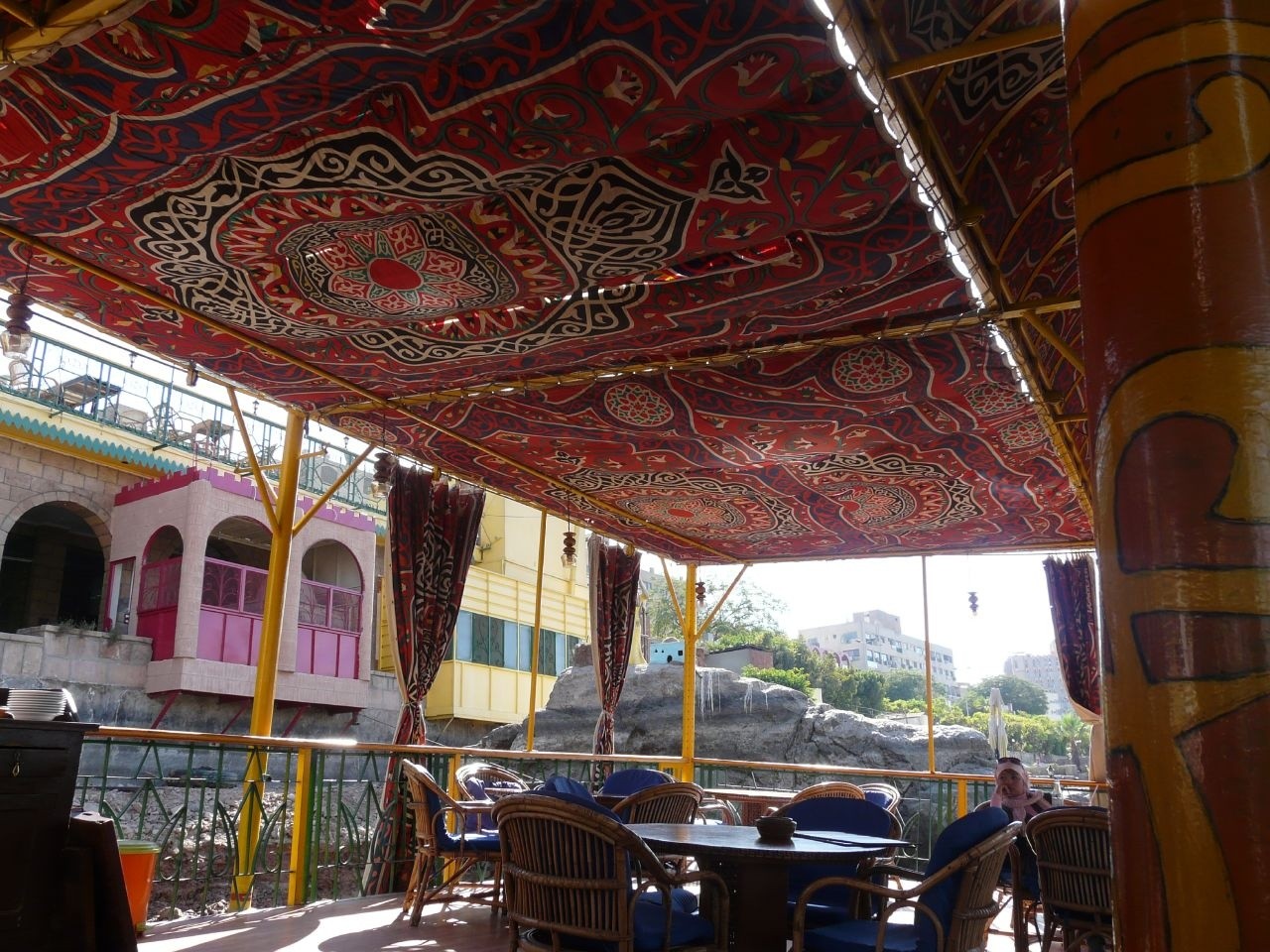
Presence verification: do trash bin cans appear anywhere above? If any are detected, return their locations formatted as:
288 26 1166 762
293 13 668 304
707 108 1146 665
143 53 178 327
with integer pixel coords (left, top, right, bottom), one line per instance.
117 839 161 936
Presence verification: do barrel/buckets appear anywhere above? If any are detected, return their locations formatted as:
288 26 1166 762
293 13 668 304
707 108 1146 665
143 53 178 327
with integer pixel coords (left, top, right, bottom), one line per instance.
117 839 162 937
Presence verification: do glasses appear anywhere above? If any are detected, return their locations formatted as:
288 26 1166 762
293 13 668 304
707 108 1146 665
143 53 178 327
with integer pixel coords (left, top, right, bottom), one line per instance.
995 757 1024 773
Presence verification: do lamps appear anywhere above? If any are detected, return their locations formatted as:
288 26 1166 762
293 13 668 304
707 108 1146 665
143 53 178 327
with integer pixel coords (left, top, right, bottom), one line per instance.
0 244 39 358
695 553 709 611
968 591 980 617
562 493 578 568
372 412 392 496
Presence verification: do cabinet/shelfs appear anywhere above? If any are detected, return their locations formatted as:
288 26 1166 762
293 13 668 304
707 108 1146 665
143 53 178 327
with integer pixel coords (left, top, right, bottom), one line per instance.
0 719 100 952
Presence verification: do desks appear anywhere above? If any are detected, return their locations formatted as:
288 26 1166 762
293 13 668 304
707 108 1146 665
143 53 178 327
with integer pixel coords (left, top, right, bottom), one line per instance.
702 788 798 825
622 822 888 952
191 420 234 463
483 785 629 809
45 374 121 423
101 404 148 433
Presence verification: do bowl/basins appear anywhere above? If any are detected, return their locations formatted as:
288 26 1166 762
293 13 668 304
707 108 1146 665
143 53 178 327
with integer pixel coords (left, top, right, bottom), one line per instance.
0 690 67 721
755 816 797 841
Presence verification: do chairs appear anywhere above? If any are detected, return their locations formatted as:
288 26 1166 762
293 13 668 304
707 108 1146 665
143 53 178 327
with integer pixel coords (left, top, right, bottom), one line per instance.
399 758 1114 952
8 359 197 452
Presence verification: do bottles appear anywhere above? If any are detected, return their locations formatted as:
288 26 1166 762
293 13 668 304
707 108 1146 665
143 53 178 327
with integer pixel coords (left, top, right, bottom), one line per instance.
1051 777 1064 808
1079 793 1092 806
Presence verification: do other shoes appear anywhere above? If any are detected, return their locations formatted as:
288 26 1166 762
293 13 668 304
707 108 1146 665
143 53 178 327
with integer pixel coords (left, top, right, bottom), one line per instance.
1086 936 1106 952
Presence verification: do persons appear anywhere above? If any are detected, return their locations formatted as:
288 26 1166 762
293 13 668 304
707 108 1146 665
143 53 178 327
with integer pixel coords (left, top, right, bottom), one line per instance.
990 757 1052 901
